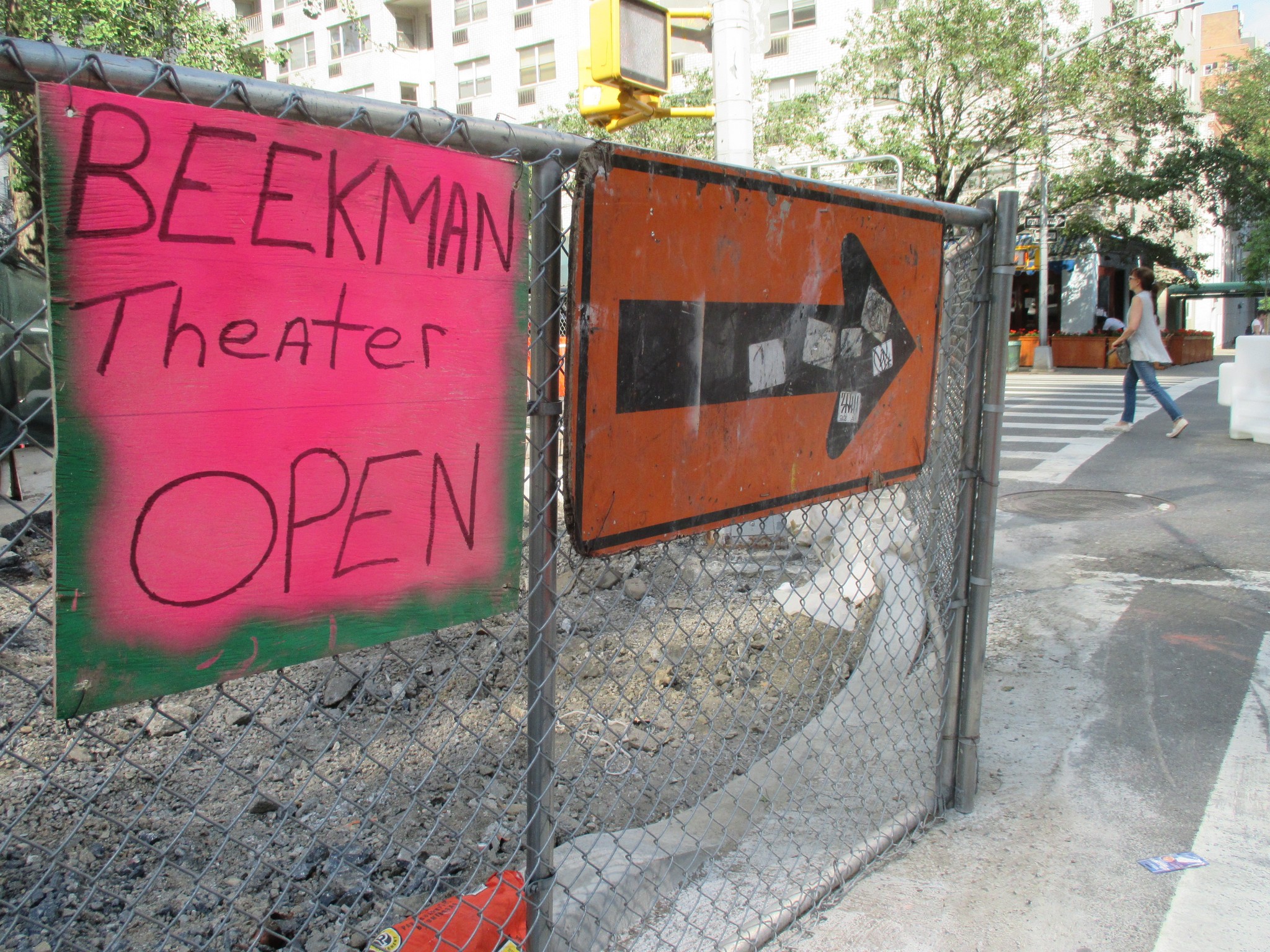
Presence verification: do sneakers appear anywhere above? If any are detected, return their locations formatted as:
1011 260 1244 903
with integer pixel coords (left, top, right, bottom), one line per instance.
1104 425 1130 432
1166 418 1188 438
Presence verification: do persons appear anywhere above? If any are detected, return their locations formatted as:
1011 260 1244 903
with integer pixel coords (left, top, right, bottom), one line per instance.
1251 310 1267 336
1097 315 1126 333
1104 266 1189 438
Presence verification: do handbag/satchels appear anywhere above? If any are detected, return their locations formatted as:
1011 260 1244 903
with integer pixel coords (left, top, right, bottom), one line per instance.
1107 343 1133 364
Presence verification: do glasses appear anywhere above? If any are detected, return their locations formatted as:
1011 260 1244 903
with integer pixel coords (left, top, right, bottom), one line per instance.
1128 275 1136 281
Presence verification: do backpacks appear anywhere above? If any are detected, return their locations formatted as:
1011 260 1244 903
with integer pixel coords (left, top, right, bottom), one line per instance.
1245 325 1252 336
